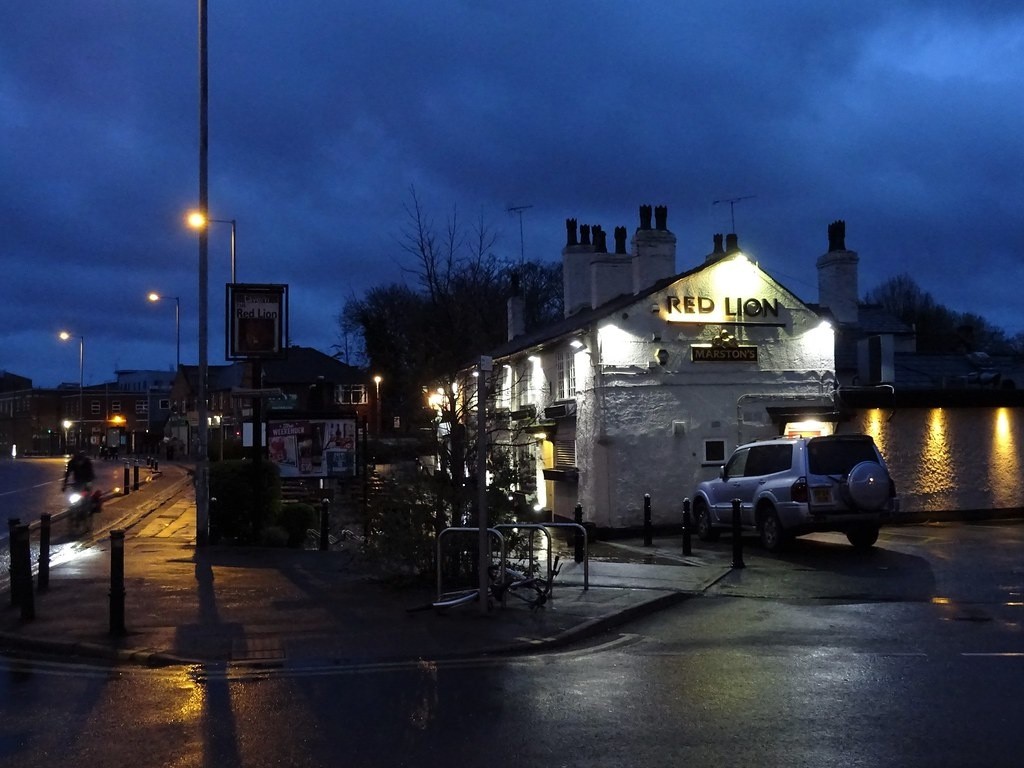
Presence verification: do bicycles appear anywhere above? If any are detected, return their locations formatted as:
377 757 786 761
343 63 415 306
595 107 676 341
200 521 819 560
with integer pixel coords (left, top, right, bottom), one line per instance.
404 554 564 616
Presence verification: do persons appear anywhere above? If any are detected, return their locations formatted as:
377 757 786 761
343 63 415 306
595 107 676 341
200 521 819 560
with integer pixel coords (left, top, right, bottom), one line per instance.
62 449 95 521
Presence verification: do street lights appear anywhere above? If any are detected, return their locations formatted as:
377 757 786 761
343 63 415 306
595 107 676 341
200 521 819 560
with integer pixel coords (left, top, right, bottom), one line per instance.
184 210 254 294
150 293 181 373
60 331 84 447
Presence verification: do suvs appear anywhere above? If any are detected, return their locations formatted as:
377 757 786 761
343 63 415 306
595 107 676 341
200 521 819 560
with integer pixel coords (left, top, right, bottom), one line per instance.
694 431 900 548
